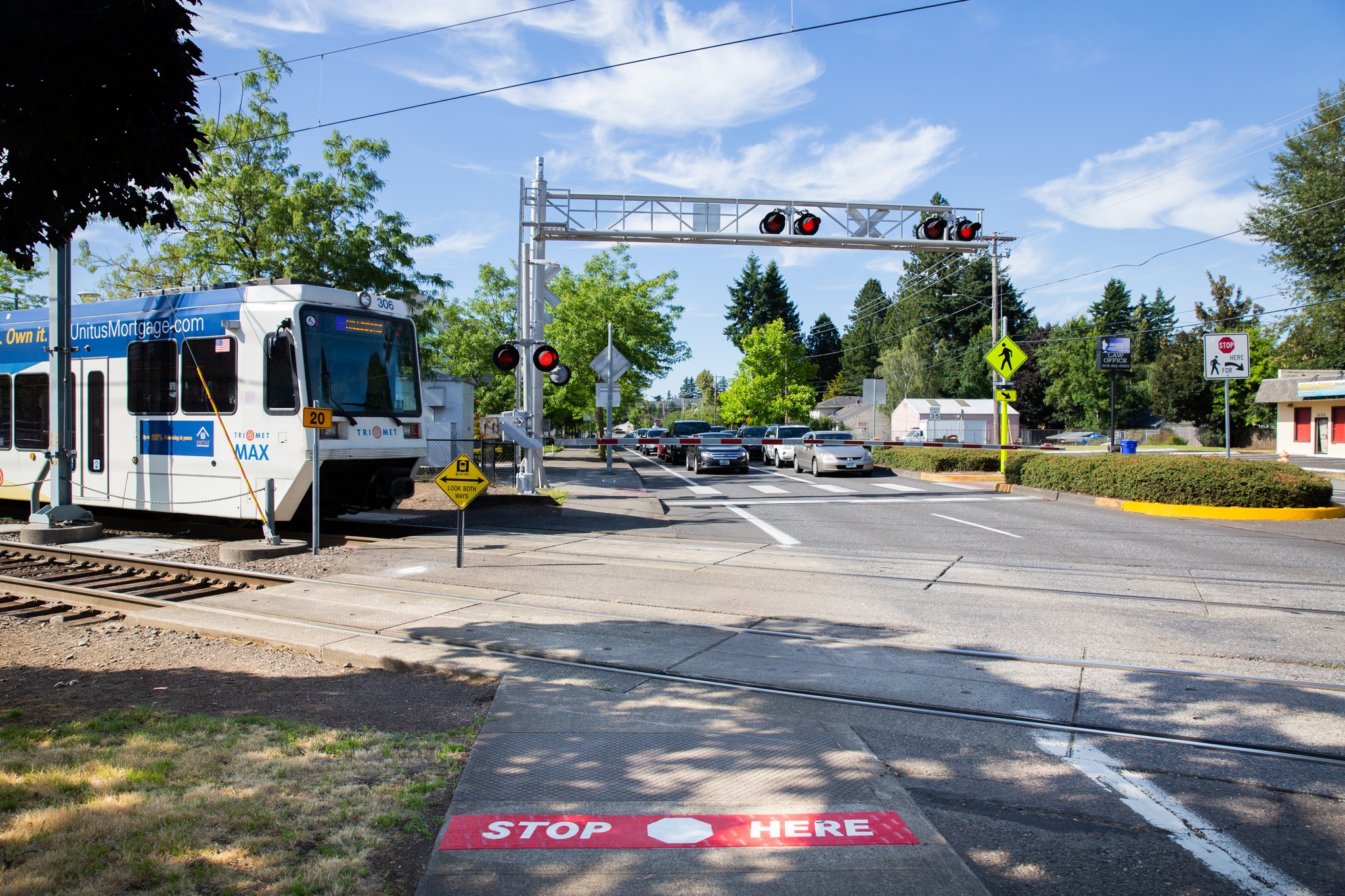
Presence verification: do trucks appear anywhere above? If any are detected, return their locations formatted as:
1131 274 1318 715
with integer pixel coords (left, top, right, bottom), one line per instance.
899 419 987 444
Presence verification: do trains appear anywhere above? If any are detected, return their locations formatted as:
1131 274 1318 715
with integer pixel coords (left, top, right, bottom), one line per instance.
0 276 429 524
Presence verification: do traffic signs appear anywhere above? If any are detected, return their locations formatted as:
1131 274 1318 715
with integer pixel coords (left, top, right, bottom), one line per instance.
995 390 1017 401
434 452 491 510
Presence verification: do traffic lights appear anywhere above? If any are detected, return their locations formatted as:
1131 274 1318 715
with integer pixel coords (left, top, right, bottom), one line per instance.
538 350 553 367
961 224 973 238
498 350 515 365
802 218 815 231
928 222 940 236
768 216 782 231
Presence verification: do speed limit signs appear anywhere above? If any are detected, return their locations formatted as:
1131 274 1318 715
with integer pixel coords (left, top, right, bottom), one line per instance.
929 407 940 420
303 407 332 429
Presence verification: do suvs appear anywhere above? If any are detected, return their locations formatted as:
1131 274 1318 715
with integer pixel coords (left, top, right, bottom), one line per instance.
761 424 813 468
664 420 713 465
633 429 648 450
710 425 728 433
735 426 769 460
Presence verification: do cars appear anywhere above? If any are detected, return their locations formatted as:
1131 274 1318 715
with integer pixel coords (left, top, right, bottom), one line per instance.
639 428 649 452
629 430 636 433
612 429 623 432
657 431 669 460
686 432 749 474
792 430 873 477
623 433 636 446
642 428 669 455
720 430 738 437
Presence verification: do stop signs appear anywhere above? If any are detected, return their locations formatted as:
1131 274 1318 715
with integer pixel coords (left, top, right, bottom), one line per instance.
1203 333 1250 380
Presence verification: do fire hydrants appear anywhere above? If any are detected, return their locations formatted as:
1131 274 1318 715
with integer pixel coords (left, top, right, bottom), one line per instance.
1277 450 1290 465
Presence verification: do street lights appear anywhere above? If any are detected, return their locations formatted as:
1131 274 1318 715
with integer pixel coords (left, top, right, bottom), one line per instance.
943 293 1000 445
